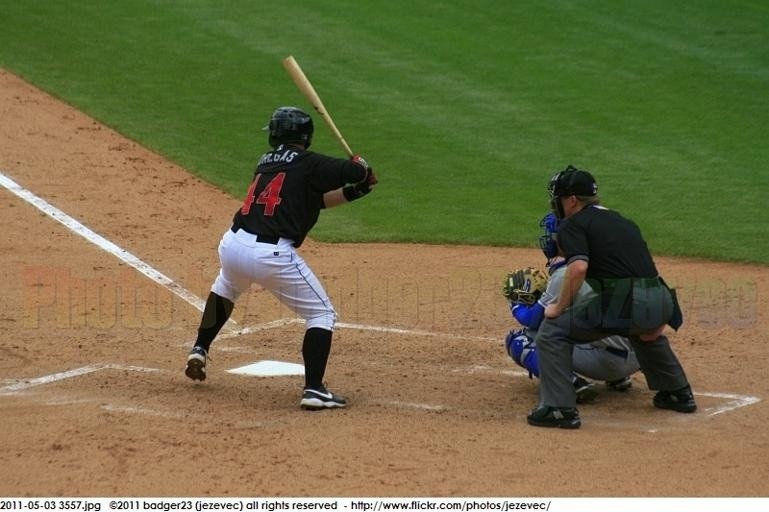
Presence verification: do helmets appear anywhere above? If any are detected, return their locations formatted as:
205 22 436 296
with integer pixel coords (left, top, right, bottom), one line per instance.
547 164 598 221
259 105 315 151
537 212 564 260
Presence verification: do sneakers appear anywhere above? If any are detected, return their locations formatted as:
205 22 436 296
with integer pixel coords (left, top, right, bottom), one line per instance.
571 371 599 402
602 376 634 390
184 345 208 382
524 403 582 430
299 382 349 411
650 382 699 415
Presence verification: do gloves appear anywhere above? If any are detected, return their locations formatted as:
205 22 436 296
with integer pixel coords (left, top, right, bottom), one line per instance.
350 154 377 197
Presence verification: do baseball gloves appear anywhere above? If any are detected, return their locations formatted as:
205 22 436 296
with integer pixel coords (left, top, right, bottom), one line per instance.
503 266 550 306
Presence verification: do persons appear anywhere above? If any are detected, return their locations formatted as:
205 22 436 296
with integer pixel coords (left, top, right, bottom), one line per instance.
185 104 376 411
501 209 643 407
526 165 700 428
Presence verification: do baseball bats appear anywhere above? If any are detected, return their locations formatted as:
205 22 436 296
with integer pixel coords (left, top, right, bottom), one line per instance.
281 56 355 160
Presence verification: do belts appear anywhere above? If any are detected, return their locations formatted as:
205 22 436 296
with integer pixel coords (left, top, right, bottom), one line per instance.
230 222 281 246
595 276 661 292
